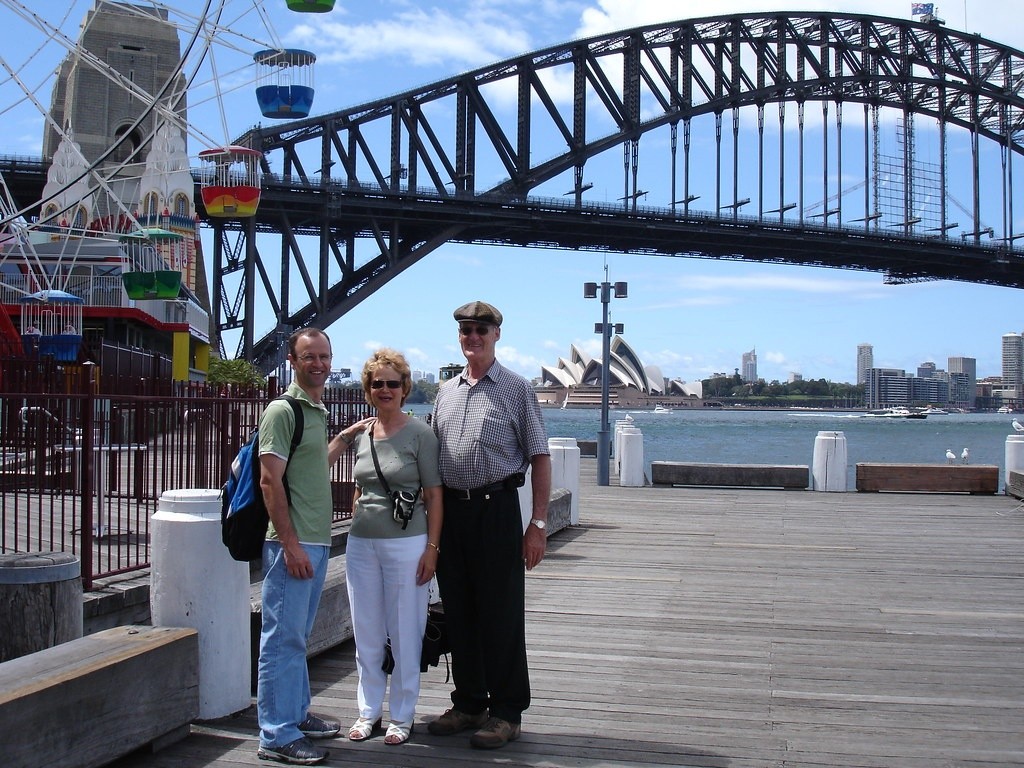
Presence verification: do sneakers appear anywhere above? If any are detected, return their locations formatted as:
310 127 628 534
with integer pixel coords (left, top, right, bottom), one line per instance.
428 707 488 735
471 716 522 748
257 737 329 765
298 712 340 738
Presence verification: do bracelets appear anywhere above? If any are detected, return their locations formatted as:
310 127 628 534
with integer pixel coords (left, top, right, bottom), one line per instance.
428 542 441 553
340 431 353 446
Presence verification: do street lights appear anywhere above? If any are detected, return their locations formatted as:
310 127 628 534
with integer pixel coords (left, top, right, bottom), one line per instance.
592 310 625 430
582 264 629 486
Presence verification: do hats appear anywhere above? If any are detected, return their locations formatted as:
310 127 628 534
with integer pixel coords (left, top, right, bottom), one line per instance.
453 301 503 327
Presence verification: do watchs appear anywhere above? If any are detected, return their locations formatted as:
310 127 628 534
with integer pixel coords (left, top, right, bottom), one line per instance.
530 519 546 530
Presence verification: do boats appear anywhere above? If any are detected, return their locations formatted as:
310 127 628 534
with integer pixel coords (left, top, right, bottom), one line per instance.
860 405 949 419
997 405 1013 414
624 414 635 423
653 404 674 414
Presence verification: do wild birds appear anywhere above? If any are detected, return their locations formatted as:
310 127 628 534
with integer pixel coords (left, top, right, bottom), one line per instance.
945 448 970 466
1010 418 1024 435
625 414 634 423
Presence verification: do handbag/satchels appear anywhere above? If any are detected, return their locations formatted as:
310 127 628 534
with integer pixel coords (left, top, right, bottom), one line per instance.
391 491 416 523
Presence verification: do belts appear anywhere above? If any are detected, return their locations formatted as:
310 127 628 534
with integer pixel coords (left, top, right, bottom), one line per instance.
444 482 511 501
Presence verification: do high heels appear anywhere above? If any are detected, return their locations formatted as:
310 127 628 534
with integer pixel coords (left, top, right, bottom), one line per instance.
349 717 382 740
384 719 414 744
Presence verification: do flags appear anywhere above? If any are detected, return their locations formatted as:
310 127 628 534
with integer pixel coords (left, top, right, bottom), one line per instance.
912 3 934 15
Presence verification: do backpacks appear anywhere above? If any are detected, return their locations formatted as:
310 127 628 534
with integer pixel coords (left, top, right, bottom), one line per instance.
217 394 305 562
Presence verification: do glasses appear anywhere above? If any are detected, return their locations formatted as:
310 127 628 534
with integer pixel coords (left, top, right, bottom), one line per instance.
371 380 404 389
297 353 330 364
459 327 495 335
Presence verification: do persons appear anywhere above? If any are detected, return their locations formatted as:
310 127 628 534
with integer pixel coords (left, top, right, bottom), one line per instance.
345 301 552 751
257 329 376 765
